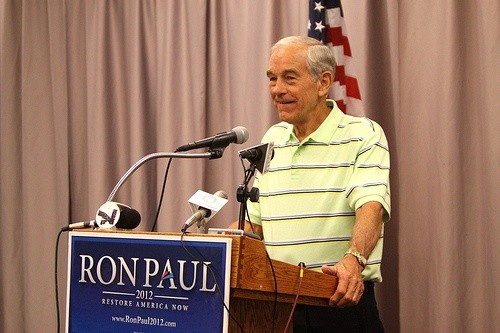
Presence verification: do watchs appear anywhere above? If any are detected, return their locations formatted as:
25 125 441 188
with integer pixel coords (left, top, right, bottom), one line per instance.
344 250 367 272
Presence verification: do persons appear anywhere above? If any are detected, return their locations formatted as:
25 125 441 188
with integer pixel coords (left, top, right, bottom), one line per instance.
221 34 391 333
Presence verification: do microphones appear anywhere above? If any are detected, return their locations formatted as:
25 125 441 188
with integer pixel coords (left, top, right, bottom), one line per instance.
238 142 275 174
177 126 249 152
181 189 230 232
62 201 141 231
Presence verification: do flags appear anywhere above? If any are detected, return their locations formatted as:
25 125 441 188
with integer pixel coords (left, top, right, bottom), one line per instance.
307 0 362 121
